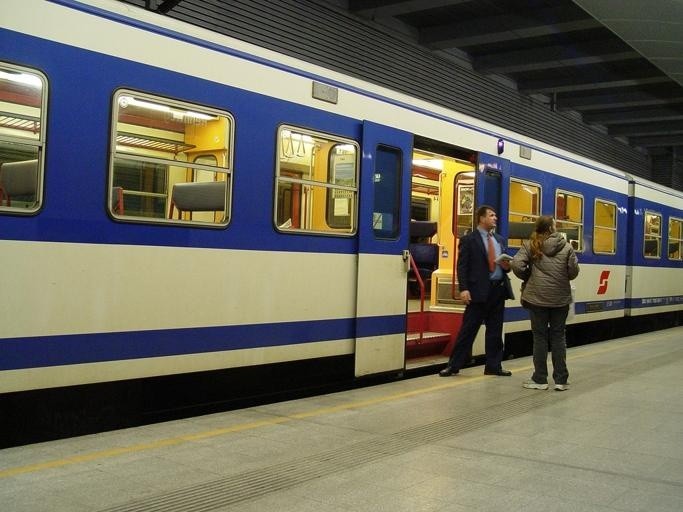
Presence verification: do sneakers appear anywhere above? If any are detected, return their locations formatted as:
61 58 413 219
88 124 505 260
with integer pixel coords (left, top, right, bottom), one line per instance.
522 378 549 390
554 379 573 390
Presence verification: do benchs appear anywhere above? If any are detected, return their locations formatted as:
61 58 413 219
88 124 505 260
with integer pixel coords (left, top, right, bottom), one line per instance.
169 181 225 223
669 244 676 253
645 239 657 256
112 187 125 215
556 229 578 242
408 221 439 300
509 221 536 239
0 159 38 207
457 226 472 235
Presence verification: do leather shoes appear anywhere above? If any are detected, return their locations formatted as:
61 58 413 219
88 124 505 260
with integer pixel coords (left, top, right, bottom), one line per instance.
439 366 460 376
484 369 512 376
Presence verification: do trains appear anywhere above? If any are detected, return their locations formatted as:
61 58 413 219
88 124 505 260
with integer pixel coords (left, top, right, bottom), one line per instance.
0 1 683 449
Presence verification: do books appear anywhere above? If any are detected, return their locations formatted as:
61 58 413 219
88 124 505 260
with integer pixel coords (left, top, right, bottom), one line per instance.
491 252 514 265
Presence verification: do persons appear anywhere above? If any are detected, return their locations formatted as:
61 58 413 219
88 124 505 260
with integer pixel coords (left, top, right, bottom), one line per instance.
507 214 581 391
436 206 516 378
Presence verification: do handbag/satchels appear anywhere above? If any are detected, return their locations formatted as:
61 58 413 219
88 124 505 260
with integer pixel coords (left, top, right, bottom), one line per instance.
520 282 531 311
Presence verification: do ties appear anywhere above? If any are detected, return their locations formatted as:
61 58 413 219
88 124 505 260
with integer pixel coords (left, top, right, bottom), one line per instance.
486 233 496 273
